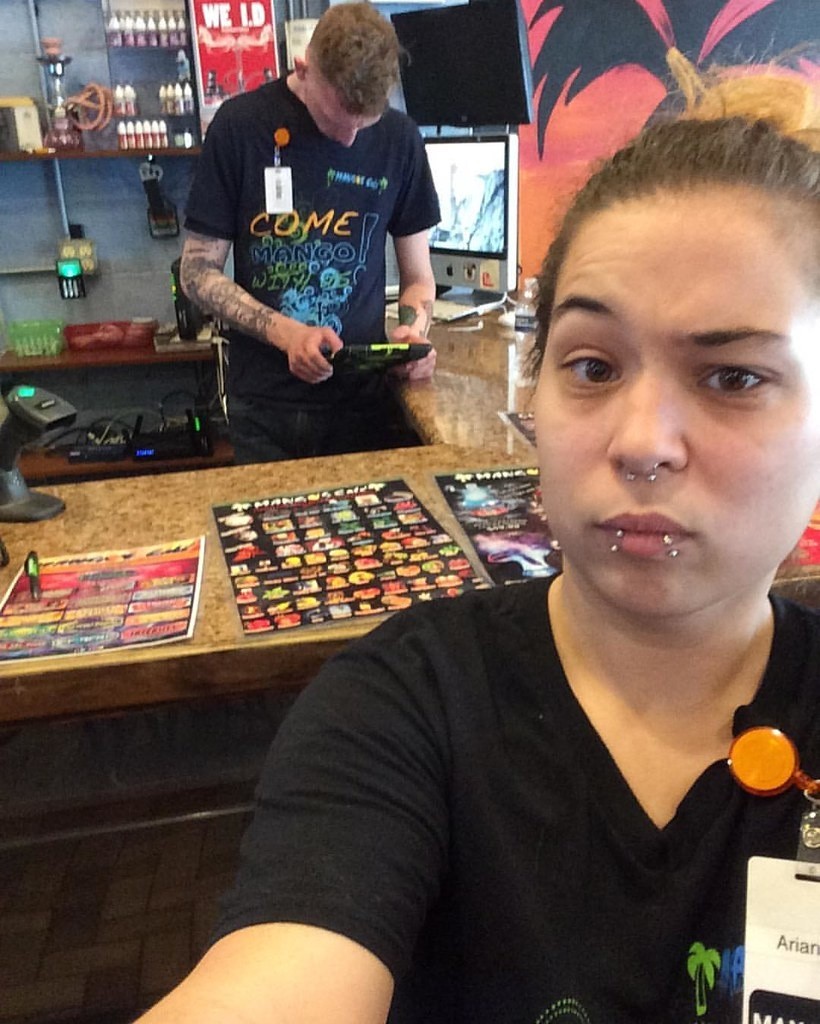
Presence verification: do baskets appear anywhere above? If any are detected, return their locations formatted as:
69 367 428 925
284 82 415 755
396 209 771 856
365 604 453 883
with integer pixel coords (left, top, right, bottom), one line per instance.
11 319 61 357
63 320 160 353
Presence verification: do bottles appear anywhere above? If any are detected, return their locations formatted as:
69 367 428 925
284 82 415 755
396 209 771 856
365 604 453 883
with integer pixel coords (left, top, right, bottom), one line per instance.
171 257 205 341
107 9 201 148
514 278 542 379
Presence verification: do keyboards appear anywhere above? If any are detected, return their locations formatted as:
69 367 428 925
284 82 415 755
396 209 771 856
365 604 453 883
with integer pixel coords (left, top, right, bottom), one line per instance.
385 299 477 322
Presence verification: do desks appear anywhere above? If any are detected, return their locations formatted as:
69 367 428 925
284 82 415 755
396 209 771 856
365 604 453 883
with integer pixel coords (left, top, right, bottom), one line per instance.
0 440 820 976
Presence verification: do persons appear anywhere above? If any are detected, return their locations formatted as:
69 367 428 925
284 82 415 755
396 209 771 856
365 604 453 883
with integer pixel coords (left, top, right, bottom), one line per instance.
179 0 440 466
473 527 564 586
127 72 820 1024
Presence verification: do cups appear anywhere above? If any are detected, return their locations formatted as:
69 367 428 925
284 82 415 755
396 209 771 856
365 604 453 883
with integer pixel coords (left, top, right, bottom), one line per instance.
185 426 214 456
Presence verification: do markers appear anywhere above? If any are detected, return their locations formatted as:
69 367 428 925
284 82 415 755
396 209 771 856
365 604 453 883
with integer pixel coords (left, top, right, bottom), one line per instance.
22 548 41 602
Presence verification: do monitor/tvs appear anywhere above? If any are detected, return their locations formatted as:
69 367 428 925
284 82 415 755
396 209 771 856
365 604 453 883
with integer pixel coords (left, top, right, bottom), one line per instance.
391 0 533 129
416 131 520 321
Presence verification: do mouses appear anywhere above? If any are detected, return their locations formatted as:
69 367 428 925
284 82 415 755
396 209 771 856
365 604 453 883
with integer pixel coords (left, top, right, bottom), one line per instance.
498 311 517 327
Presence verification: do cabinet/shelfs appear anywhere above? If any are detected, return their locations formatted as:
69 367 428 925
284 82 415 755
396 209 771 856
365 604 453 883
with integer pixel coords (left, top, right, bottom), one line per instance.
98 0 203 155
0 336 231 481
